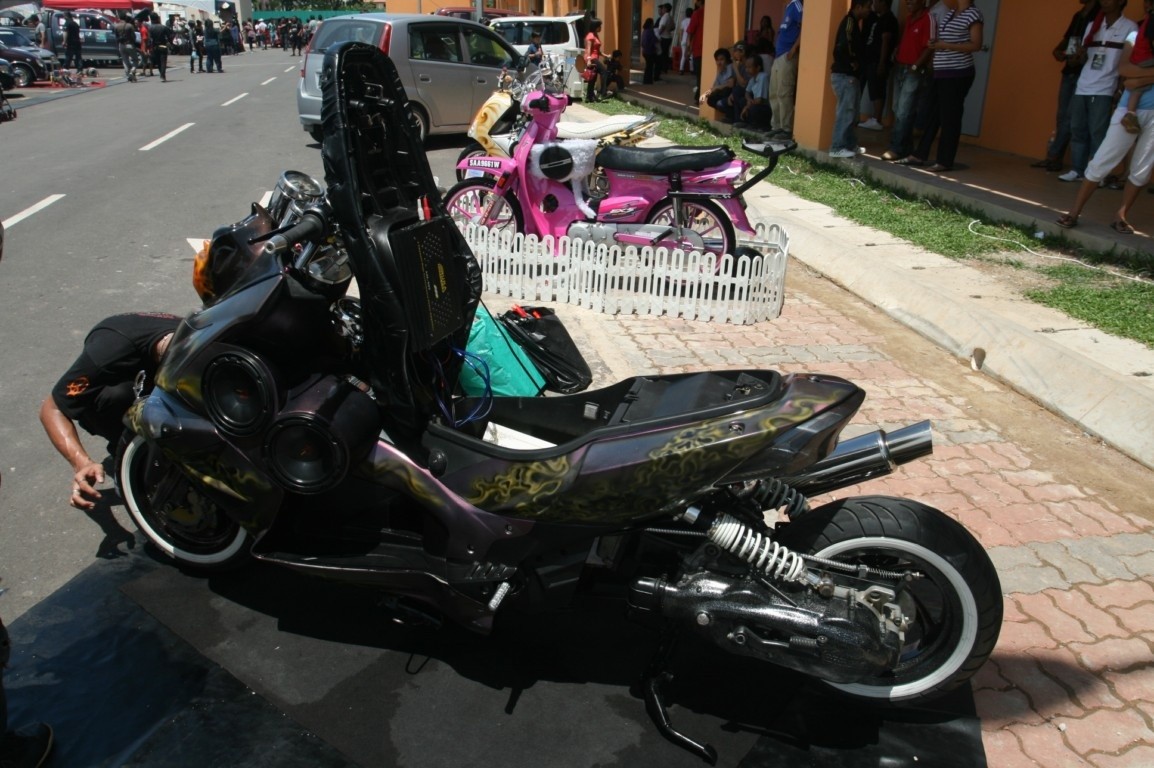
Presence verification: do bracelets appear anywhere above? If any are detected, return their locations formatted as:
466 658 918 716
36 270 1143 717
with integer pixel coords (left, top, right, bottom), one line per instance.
586 56 591 59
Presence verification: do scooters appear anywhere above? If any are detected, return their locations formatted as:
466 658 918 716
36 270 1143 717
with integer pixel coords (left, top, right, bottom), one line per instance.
439 48 798 293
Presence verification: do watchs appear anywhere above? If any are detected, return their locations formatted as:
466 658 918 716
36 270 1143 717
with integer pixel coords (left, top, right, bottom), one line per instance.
910 63 919 71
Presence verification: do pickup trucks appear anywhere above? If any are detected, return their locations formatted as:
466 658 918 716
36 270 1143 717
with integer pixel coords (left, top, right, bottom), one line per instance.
0 10 146 69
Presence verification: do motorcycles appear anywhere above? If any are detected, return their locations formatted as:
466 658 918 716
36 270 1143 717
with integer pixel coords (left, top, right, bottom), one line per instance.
115 40 1007 768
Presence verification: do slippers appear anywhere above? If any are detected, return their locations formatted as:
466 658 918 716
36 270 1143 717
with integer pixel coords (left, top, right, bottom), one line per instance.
1056 214 1077 228
925 163 953 171
1110 220 1133 234
894 155 924 165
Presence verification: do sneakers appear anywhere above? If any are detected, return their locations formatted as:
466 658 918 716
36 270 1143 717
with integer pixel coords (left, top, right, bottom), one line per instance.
851 147 866 154
1098 179 1106 188
829 147 856 157
1059 170 1084 181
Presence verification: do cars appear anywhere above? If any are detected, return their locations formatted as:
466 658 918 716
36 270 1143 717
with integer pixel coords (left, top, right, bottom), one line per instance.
1 27 61 92
297 11 523 146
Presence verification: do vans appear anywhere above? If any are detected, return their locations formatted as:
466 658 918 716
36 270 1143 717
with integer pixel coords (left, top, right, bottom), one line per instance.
434 7 532 27
487 14 590 101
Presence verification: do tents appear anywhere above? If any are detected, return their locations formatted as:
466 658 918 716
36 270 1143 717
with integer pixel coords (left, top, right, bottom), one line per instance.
0 0 153 19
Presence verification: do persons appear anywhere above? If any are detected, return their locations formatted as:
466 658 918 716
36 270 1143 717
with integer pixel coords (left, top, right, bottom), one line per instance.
470 16 506 59
641 0 705 93
12 17 32 27
87 13 323 83
699 0 982 172
61 11 83 76
527 32 544 65
40 312 187 514
583 18 626 104
31 14 53 72
1045 0 1153 234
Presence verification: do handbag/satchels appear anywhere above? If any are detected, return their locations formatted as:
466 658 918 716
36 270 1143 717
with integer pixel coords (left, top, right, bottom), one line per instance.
582 68 596 81
460 305 592 398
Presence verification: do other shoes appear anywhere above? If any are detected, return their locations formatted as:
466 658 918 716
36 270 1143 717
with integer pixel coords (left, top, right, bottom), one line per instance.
1112 177 1124 190
1032 159 1060 167
720 116 735 124
690 71 696 75
882 150 901 160
775 131 791 139
0 721 53 768
857 117 883 131
1121 113 1141 133
765 130 781 137
678 70 685 75
733 122 748 128
